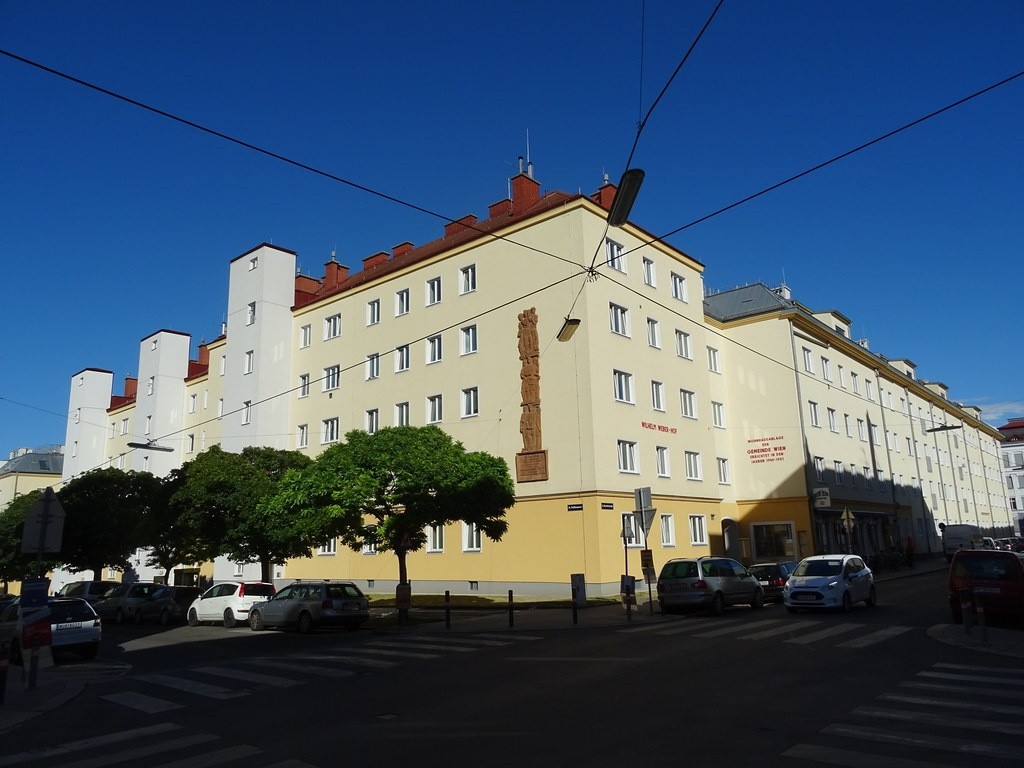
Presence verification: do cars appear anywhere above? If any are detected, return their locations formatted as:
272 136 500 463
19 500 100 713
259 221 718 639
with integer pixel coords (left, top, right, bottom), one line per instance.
94 583 163 627
186 581 276 628
948 533 1024 569
746 559 798 604
656 555 765 614
246 579 369 634
947 548 1024 626
135 586 203 625
782 554 877 615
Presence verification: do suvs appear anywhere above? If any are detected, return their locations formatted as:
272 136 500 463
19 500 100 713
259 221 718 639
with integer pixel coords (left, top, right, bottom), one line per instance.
1 596 103 666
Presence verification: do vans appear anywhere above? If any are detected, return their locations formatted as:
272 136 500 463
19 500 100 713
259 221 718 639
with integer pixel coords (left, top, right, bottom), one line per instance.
55 581 118 608
